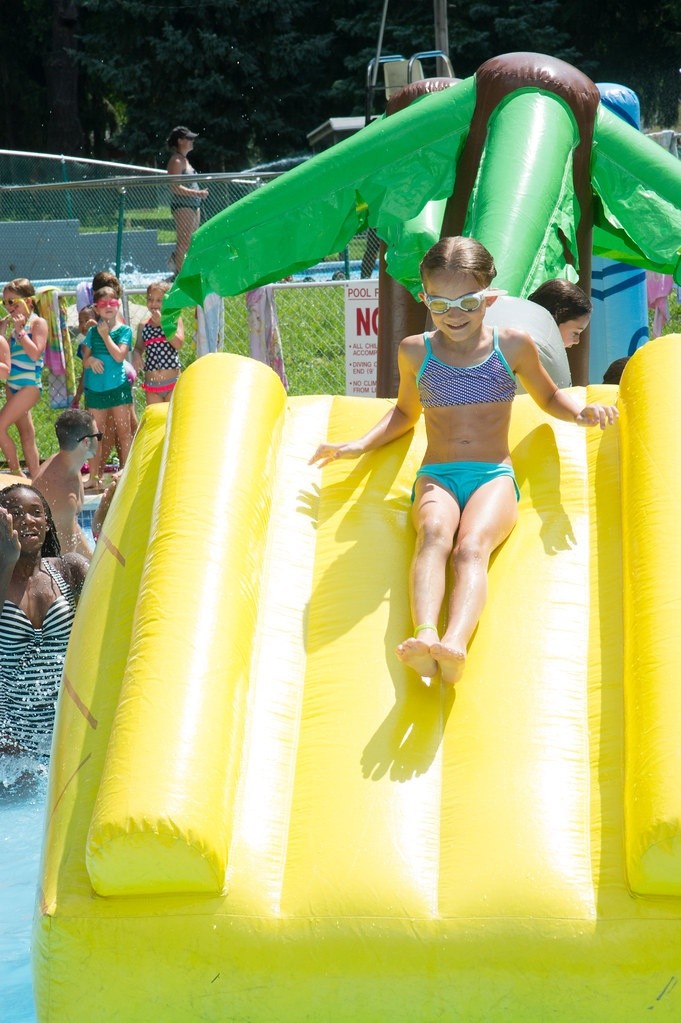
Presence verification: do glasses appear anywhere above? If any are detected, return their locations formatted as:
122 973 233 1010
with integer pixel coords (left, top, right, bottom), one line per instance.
95 299 119 308
422 282 492 313
2 296 36 305
76 431 103 443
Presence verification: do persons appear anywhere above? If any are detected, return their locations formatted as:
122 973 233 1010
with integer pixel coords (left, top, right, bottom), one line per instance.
0 270 185 488
167 126 208 282
308 235 619 684
33 408 103 563
360 227 381 280
0 484 90 805
527 278 592 349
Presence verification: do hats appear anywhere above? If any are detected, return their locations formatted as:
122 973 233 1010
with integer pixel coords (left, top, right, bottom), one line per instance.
169 126 198 146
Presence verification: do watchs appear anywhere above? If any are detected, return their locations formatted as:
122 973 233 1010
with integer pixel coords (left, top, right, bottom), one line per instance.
16 332 26 340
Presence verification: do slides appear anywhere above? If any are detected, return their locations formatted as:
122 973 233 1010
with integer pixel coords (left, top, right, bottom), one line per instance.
36 328 681 1023
